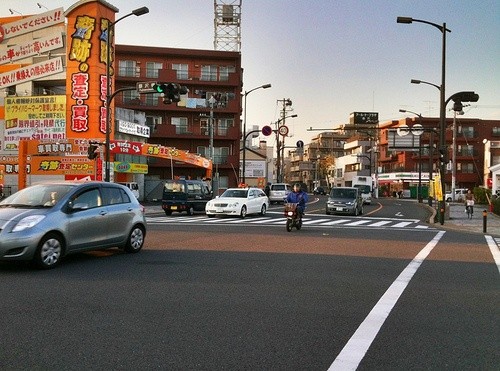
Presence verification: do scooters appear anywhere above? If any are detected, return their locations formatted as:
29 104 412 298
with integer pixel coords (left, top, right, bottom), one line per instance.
282 195 303 232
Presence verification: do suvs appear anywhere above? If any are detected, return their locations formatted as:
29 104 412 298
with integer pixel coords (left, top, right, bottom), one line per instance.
445 187 468 202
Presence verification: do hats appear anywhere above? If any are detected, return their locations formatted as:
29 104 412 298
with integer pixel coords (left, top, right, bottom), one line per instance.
294 183 300 187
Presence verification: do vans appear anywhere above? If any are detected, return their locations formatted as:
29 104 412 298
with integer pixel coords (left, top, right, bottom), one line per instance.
161 179 215 216
325 186 363 216
269 183 293 205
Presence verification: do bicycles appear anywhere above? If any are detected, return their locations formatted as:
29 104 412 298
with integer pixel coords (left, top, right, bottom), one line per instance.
466 200 474 220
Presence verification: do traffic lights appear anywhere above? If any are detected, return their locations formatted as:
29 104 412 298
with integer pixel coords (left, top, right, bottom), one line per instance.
90 146 98 158
153 82 180 93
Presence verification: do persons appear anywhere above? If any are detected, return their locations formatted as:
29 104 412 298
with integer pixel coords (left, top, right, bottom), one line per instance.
464 189 474 216
284 183 308 219
265 183 271 198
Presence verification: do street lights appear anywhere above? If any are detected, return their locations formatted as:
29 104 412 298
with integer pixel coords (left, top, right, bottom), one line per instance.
105 5 149 184
351 154 371 177
277 114 297 183
242 84 272 185
410 78 445 224
394 15 452 226
398 109 423 203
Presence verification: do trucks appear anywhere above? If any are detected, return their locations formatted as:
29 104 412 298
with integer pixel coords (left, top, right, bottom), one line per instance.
352 176 373 205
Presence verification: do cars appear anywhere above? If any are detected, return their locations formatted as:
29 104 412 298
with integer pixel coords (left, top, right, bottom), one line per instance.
205 186 270 218
0 179 148 271
312 186 326 195
